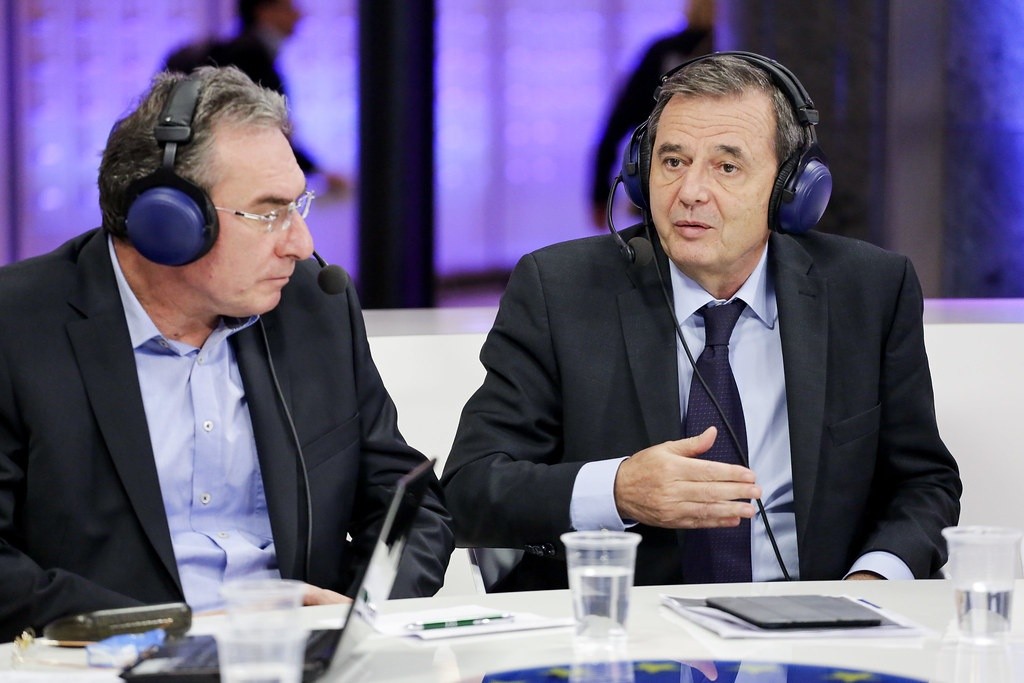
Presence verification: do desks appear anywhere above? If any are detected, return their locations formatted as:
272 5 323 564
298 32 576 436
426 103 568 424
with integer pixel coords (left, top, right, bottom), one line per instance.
0 580 1024 683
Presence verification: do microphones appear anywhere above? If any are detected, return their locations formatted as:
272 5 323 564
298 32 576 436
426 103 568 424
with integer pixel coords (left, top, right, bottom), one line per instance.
605 173 654 266
313 250 350 295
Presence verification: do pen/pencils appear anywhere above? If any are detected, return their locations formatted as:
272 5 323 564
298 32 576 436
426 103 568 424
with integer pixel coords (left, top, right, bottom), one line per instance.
406 613 515 630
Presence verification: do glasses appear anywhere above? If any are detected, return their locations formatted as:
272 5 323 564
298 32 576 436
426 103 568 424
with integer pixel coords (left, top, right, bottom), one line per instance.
214 190 315 235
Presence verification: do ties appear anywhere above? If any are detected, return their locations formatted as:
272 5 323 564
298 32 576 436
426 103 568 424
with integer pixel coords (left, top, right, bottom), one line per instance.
685 299 754 584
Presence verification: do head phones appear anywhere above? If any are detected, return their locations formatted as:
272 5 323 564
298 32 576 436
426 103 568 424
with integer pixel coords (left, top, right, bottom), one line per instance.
619 50 833 233
122 77 221 265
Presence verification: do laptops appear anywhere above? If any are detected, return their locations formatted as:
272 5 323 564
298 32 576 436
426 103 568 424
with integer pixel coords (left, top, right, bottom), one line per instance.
118 458 438 683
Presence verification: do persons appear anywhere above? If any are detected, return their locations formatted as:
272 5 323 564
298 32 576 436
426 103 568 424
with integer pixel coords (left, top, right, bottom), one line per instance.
436 56 963 591
163 0 349 206
591 0 720 230
1 64 456 644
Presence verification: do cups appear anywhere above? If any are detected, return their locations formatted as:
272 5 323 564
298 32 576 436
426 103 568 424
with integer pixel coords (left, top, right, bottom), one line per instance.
560 531 641 637
210 620 311 683
942 527 1023 645
217 580 302 621
